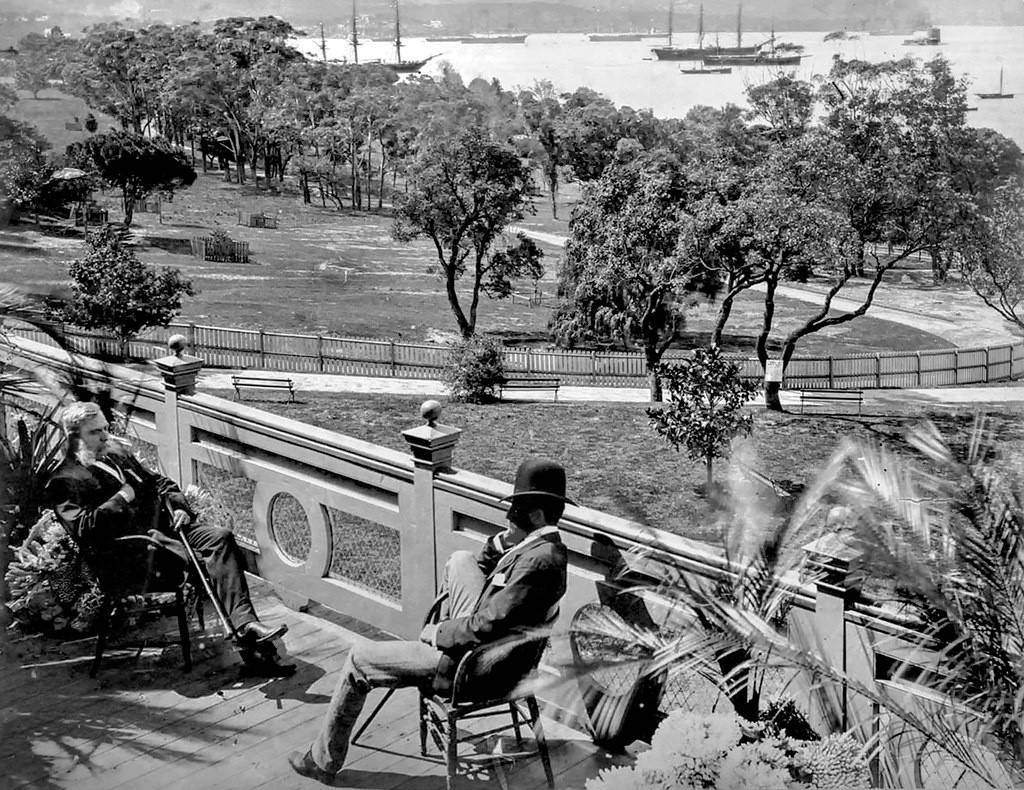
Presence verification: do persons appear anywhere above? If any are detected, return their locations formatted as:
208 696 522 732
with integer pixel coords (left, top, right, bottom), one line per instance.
288 460 580 784
44 402 297 679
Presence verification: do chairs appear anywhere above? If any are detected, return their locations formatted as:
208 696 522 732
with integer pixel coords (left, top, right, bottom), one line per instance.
419 593 561 790
45 478 206 679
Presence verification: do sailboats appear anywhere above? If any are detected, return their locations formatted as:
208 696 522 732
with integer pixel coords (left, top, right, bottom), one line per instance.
973 65 1014 98
313 0 443 72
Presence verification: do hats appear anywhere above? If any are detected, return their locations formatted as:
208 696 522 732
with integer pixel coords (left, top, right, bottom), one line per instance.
497 459 578 509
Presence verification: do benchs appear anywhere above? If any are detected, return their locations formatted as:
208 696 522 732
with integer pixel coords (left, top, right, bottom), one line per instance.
499 378 560 402
231 375 299 407
736 458 795 511
799 389 864 415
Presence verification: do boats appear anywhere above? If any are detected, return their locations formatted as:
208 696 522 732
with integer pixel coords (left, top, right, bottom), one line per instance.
426 35 473 41
704 23 801 65
680 61 732 74
588 33 641 41
461 33 528 43
902 28 945 45
651 2 762 60
641 33 672 37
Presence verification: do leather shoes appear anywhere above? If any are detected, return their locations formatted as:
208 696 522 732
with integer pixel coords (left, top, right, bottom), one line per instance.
232 621 288 655
289 751 336 785
238 643 298 677
